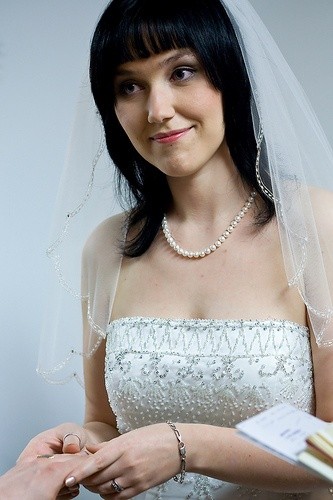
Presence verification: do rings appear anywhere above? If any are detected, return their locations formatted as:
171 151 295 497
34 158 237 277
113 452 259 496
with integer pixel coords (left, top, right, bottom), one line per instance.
84 450 89 455
63 433 80 441
112 479 123 493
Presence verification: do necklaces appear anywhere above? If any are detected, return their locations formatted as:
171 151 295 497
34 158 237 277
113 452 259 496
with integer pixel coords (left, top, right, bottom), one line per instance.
162 188 256 258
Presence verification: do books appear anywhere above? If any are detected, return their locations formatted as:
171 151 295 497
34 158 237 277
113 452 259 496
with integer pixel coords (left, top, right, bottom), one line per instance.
239 403 333 481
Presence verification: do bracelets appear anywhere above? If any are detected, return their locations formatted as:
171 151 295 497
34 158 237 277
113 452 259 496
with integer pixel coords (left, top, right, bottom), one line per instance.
166 420 187 484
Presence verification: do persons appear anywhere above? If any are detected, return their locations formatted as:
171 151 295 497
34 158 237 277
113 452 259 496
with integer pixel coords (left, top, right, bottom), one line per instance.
33 0 333 500
0 448 93 500
